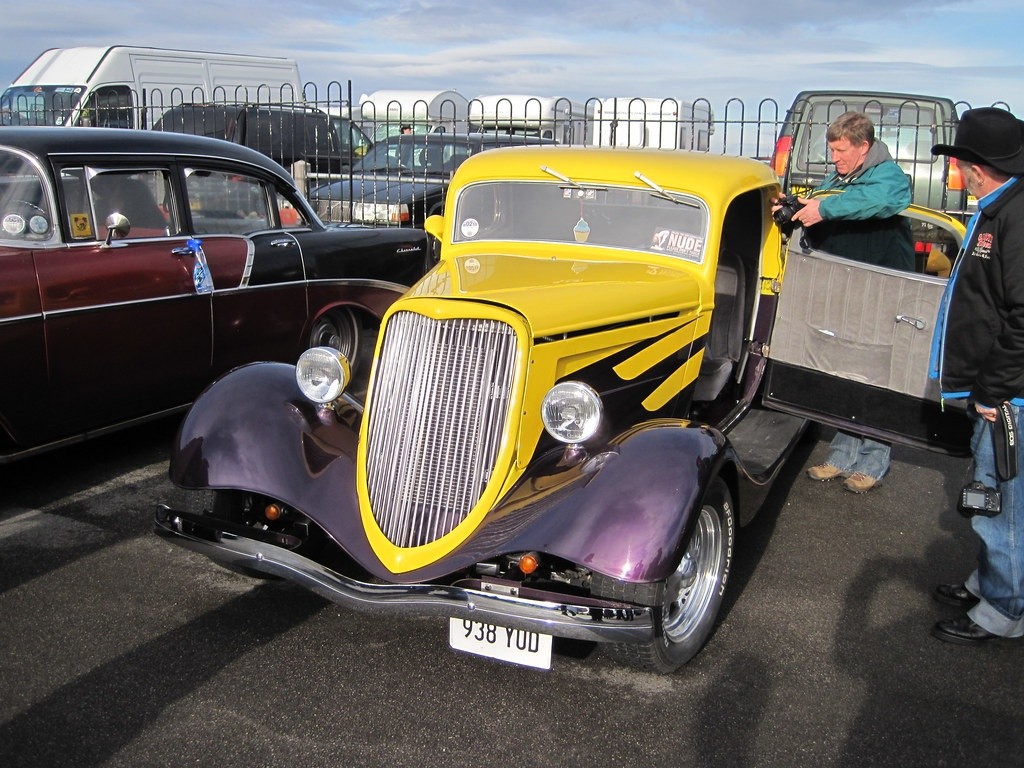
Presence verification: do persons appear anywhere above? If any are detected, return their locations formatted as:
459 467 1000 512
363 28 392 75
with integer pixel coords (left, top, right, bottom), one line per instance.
396 125 412 167
931 106 1023 640
419 127 445 168
771 112 918 491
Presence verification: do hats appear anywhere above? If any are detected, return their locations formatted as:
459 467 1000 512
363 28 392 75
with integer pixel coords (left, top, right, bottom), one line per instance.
931 108 1024 174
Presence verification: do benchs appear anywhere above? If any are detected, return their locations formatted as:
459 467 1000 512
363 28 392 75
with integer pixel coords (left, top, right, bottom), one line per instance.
193 216 266 235
96 224 168 241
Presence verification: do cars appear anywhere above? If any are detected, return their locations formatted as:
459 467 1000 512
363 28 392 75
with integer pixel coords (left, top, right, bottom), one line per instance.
310 136 562 267
0 123 439 470
151 142 971 674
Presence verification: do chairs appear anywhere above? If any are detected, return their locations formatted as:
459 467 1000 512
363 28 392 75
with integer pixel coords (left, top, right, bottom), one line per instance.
443 154 469 173
416 148 443 173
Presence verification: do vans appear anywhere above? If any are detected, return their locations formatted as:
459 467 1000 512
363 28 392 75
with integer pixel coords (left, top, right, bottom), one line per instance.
0 46 305 179
593 97 716 153
768 90 968 255
360 89 471 166
467 96 595 146
302 104 362 153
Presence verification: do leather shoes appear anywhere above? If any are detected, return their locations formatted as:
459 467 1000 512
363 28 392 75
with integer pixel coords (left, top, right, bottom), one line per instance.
935 615 1004 646
934 583 978 608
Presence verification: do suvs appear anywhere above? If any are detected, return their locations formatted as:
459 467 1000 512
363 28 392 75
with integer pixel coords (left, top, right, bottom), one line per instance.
150 101 374 190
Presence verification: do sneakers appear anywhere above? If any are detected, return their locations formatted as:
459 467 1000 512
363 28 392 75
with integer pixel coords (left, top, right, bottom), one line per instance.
807 462 854 480
843 471 877 493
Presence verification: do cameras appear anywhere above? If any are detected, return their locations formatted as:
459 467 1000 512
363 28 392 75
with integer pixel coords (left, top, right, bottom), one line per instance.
773 195 806 224
